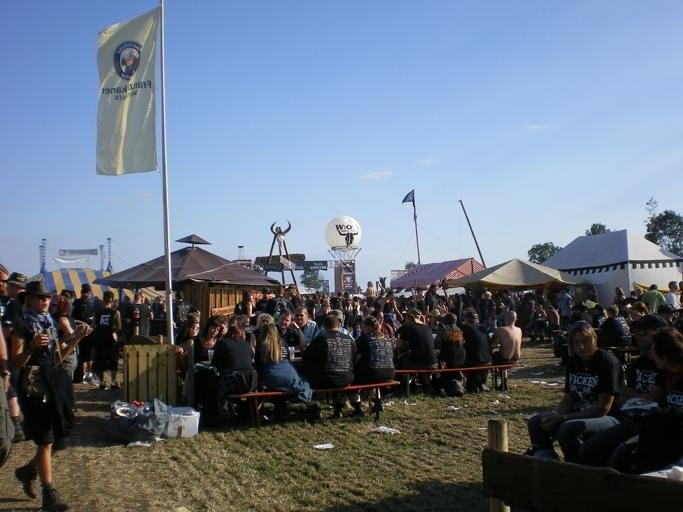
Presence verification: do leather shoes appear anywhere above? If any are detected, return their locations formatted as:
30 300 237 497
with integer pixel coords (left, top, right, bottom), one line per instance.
301 404 384 417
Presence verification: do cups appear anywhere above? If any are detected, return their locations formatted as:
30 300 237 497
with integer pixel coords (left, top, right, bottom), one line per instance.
207 349 213 363
287 346 294 360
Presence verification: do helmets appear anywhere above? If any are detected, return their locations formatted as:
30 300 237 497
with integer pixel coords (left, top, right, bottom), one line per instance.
16 280 54 305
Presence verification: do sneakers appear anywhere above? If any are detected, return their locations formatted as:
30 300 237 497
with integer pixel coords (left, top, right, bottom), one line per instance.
40 484 75 512
467 383 508 394
81 373 120 392
12 423 24 443
15 462 38 499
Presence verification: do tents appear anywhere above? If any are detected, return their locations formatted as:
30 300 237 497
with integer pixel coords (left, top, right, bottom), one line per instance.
450 257 576 291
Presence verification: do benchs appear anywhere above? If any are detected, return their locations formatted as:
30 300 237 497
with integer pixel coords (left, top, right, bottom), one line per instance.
392 364 514 399
222 377 399 428
615 345 641 362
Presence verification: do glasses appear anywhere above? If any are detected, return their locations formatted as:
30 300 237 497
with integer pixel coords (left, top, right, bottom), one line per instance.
632 328 650 338
30 295 52 300
664 311 673 315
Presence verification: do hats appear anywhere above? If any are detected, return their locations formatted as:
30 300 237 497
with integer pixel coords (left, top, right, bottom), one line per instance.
637 314 669 329
446 313 457 322
5 272 27 289
658 304 677 317
407 307 424 325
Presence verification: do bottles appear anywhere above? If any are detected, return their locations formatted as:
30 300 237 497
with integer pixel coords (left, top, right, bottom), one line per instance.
355 325 361 337
133 308 139 326
537 312 541 320
35 328 50 345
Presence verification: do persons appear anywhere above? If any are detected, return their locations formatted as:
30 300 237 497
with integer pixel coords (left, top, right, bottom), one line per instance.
171 282 522 432
522 280 682 474
0 264 170 512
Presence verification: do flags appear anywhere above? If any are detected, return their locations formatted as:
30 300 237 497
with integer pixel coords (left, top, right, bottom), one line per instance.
95 5 162 176
401 190 414 204
